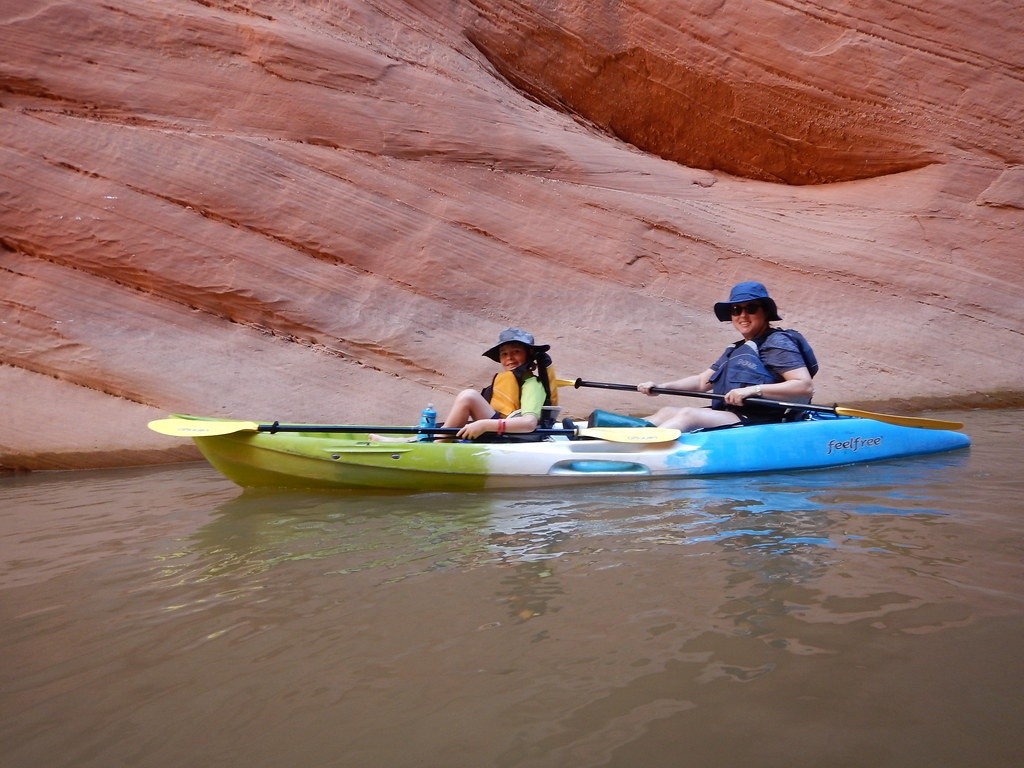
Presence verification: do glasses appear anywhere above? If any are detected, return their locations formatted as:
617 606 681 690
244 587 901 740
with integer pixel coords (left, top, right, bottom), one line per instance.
728 303 764 316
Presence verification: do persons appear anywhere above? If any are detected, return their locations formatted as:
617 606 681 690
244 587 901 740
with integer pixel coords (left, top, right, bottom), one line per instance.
368 327 551 442
562 280 815 440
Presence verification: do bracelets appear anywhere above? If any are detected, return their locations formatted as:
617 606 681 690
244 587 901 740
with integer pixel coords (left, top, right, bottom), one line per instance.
497 419 507 433
753 384 762 395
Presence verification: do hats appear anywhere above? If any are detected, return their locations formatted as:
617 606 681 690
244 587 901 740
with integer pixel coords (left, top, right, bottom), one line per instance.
714 281 782 322
482 328 551 363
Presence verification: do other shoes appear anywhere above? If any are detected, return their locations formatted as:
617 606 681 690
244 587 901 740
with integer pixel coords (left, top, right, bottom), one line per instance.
563 419 580 440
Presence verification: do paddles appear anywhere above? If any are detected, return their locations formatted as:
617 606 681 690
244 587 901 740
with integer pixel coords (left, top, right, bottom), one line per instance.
555 376 965 433
146 415 686 448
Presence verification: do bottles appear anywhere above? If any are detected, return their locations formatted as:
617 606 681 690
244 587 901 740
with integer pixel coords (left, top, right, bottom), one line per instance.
416 403 437 442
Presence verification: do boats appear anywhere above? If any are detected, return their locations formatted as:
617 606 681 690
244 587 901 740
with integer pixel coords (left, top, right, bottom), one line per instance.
167 410 971 492
